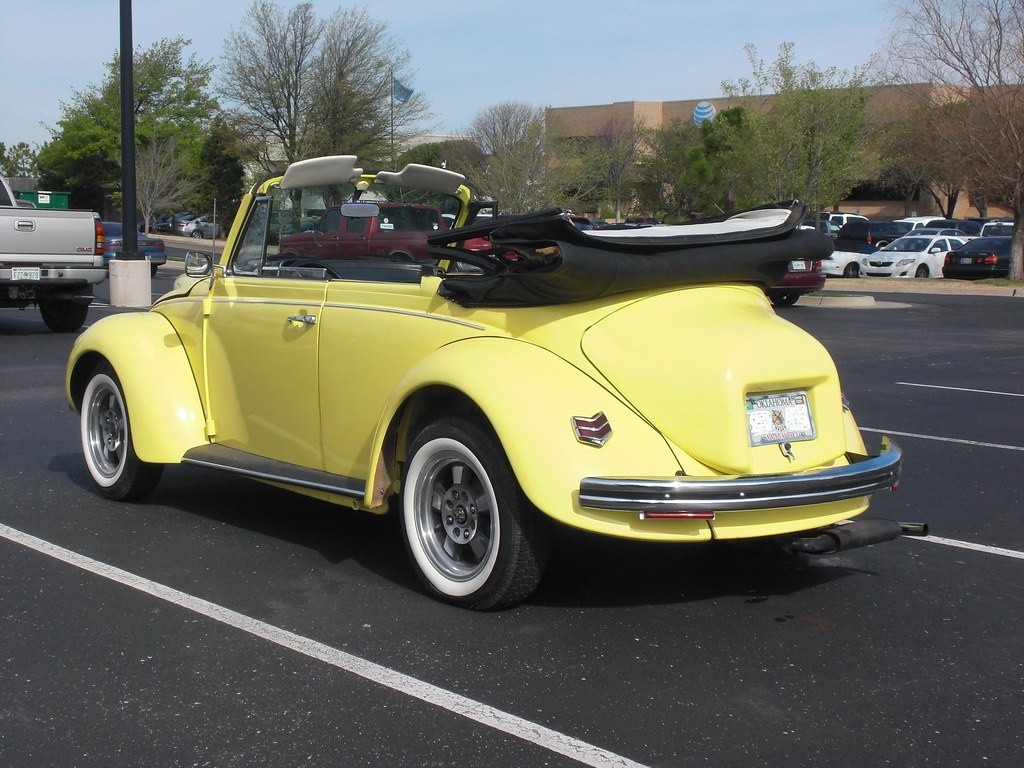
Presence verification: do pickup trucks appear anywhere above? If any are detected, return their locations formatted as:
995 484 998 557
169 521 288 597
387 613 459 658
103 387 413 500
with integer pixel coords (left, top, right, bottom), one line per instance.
279 200 520 265
0 174 107 332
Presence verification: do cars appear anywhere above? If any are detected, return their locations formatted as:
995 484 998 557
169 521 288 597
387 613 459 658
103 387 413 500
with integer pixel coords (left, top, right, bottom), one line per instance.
67 155 927 612
942 236 1013 280
102 222 167 278
858 233 966 279
441 209 1015 307
137 212 220 238
